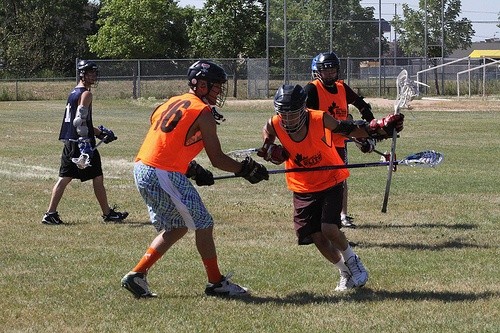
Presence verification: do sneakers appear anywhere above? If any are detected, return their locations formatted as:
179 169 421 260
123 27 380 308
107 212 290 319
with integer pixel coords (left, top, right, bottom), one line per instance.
120 268 158 299
344 254 368 287
101 204 129 222
335 270 354 292
42 211 64 225
205 271 250 298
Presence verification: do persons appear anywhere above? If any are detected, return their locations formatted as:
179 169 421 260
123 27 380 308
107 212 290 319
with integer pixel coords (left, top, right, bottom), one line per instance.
303 51 388 226
257 84 405 291
42 60 129 225
120 60 269 300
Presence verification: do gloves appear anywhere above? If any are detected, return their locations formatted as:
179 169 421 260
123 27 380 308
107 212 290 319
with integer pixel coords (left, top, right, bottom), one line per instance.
370 113 404 140
257 138 287 165
77 136 93 169
96 125 118 144
186 160 214 186
235 157 269 184
361 138 377 153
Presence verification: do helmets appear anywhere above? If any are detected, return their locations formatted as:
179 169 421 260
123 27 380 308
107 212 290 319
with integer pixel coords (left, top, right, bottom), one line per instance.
187 61 228 108
77 60 99 88
274 85 308 134
312 52 341 87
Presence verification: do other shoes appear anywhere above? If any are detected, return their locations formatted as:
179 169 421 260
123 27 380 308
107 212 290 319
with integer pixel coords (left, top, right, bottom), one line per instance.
341 215 356 228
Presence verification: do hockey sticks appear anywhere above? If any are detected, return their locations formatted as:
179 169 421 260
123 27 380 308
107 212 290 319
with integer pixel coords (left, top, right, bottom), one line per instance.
71 134 108 169
381 69 431 213
213 150 444 180
